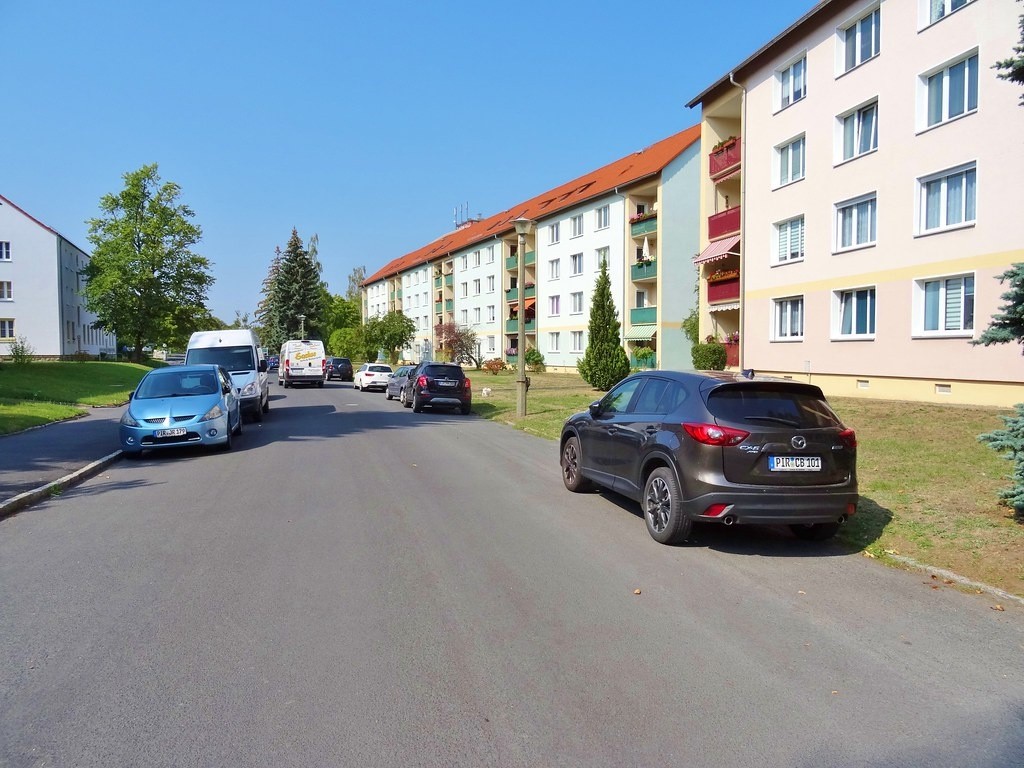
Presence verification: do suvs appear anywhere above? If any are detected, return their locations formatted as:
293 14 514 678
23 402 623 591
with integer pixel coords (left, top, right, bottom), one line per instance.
326 357 353 381
402 361 471 415
559 370 859 546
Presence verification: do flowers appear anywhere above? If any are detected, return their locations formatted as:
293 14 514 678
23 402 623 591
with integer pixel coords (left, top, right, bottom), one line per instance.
724 329 738 346
706 267 739 281
505 347 518 356
525 281 534 288
629 210 653 222
636 254 655 266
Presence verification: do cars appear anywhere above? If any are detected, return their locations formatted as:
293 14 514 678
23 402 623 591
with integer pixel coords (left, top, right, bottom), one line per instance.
118 364 242 460
354 363 394 392
265 356 279 369
385 365 417 403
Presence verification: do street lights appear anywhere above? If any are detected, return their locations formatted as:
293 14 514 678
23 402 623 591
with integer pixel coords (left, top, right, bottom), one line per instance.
508 215 536 415
299 315 306 340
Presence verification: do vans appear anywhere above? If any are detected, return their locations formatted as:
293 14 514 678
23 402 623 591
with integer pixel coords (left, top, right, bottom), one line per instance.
278 339 326 388
181 329 270 422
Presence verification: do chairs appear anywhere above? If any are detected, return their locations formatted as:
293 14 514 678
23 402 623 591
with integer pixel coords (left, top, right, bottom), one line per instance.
655 382 671 411
197 374 216 391
